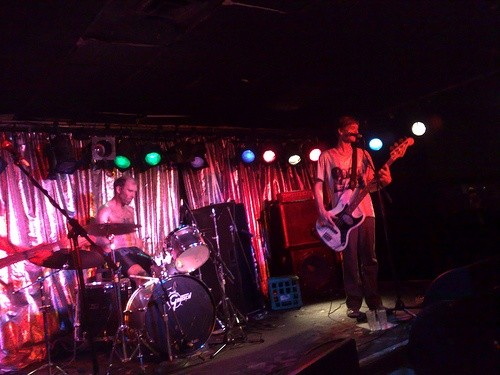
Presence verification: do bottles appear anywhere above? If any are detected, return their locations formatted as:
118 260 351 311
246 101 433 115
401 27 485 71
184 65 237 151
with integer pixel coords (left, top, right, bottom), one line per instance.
365 310 378 330
376 310 387 328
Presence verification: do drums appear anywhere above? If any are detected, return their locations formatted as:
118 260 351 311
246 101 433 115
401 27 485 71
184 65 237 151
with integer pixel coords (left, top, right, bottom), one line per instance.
149 250 191 279
163 223 211 273
73 282 124 343
124 274 218 357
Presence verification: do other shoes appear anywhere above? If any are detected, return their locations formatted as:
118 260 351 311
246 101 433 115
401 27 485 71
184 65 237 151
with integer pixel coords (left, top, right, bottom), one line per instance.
347 308 360 317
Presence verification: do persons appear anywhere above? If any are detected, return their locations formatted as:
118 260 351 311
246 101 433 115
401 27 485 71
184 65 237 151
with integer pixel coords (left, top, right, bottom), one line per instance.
95 175 163 289
314 115 394 318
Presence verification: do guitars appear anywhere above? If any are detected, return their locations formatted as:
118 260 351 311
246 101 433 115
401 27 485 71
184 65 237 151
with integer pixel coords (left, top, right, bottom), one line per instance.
315 136 415 252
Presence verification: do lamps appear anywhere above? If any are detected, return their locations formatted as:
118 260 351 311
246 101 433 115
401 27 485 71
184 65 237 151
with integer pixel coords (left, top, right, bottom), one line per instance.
48 129 209 174
237 139 321 164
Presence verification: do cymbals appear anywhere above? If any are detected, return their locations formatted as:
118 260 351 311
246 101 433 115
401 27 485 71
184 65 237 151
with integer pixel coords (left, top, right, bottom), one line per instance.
191 203 232 213
82 222 142 237
29 249 106 270
